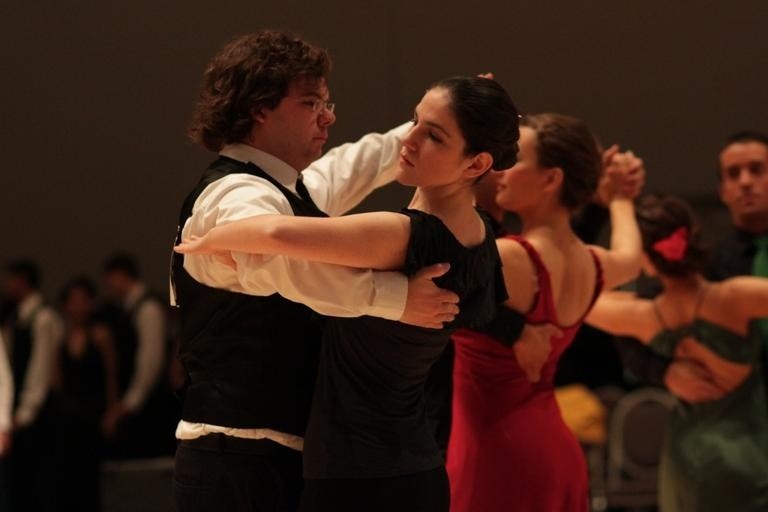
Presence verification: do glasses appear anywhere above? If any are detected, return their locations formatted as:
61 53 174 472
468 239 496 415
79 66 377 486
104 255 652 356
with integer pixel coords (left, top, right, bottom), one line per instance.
295 100 335 112
